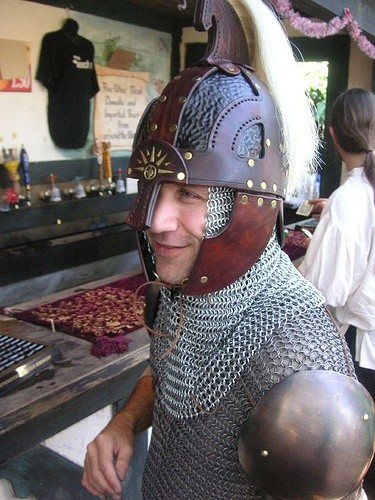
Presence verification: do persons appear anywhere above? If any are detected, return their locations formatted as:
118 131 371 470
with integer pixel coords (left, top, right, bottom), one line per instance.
36 18 100 150
296 88 375 395
80 58 375 500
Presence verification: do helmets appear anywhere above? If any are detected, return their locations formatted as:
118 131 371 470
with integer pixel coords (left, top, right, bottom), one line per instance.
127 0 288 295
